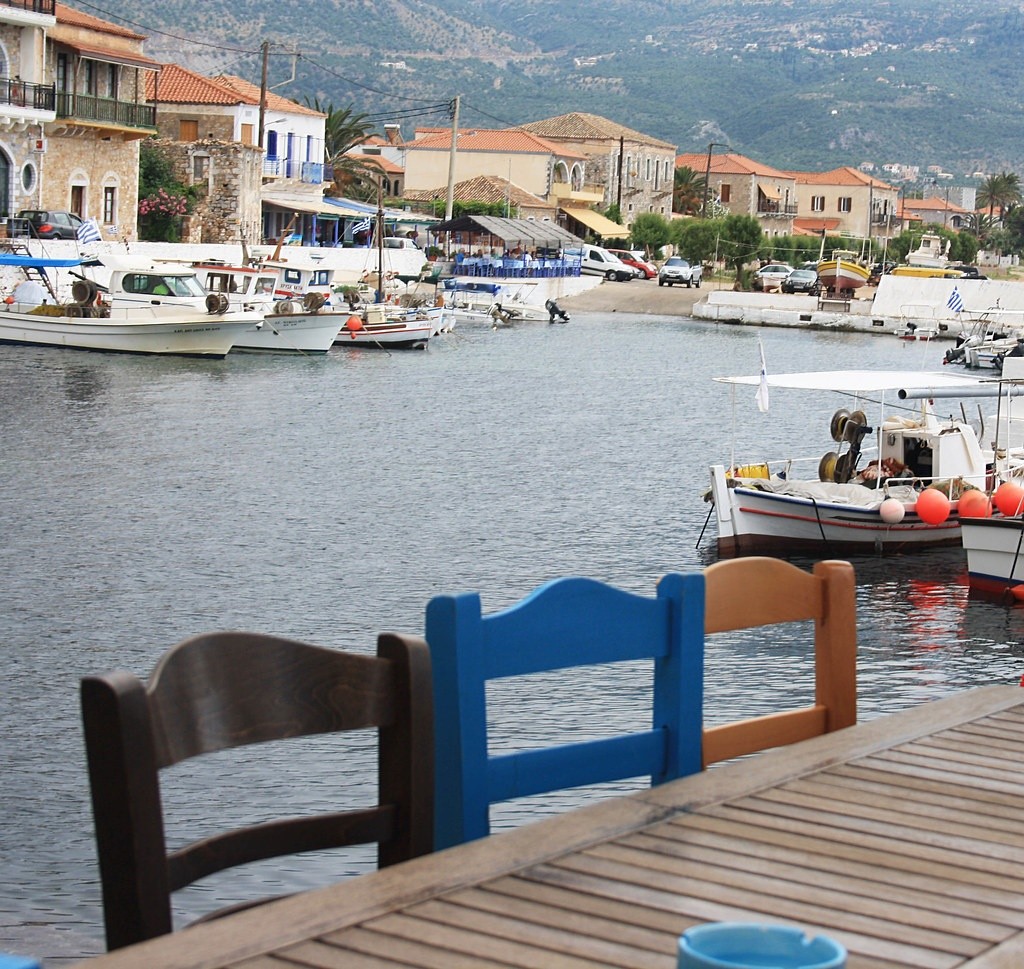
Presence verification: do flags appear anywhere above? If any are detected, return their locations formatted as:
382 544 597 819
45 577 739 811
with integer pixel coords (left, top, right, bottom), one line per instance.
76 216 100 244
946 286 964 312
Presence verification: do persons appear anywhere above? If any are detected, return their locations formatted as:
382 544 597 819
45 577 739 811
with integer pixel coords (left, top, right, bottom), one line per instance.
457 248 466 264
522 251 537 261
483 240 491 255
491 248 499 260
555 250 562 259
510 248 521 259
473 249 483 258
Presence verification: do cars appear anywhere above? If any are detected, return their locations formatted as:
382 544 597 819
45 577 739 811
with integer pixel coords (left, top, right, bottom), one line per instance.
781 269 822 296
866 261 896 285
754 264 794 291
604 248 658 280
382 236 422 250
15 209 87 241
659 256 703 288
559 243 641 282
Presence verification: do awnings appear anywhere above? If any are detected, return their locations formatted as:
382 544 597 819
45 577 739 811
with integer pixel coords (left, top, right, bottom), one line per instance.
561 207 632 240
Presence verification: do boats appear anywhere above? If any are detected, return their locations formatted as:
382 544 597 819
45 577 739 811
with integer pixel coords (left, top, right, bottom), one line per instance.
0 267 264 359
151 259 352 355
943 298 1024 369
332 302 456 349
816 256 871 292
443 302 570 320
708 400 1024 598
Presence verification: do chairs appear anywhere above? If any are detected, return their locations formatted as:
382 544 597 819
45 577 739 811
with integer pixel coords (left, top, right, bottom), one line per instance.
453 253 583 278
422 570 707 850
653 556 857 771
79 629 435 954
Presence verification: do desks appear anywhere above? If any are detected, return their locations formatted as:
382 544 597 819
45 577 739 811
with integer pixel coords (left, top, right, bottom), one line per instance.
39 684 1024 969
